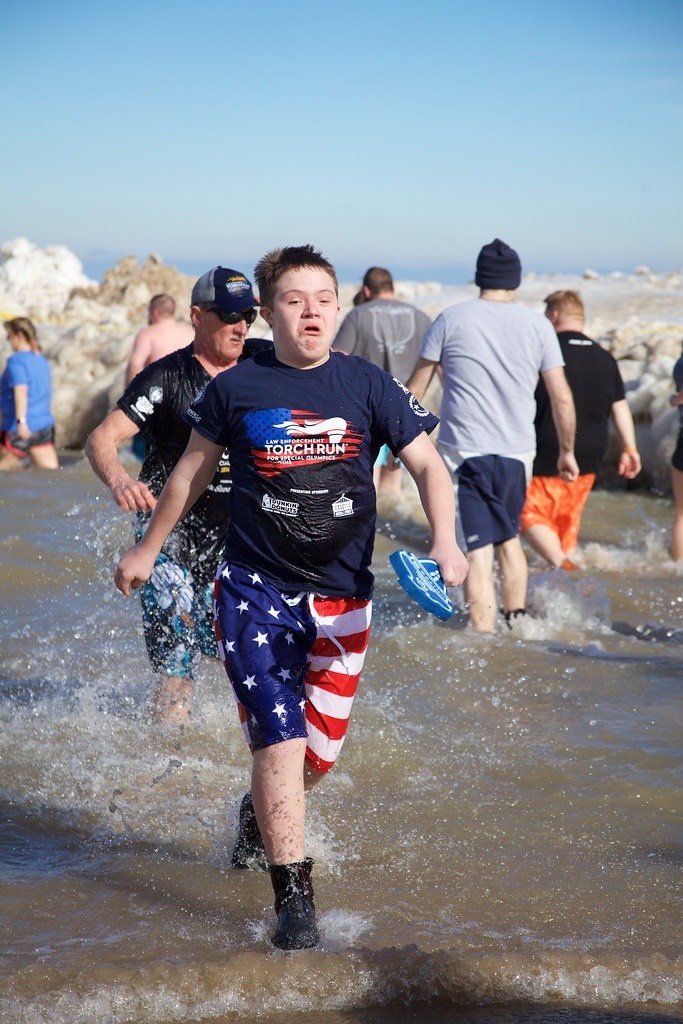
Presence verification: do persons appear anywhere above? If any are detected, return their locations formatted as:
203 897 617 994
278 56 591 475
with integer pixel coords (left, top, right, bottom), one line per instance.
669 390 683 408
113 244 470 952
80 266 263 727
352 289 369 307
515 291 644 575
664 342 683 564
0 315 64 474
328 264 452 524
403 239 582 641
125 292 198 402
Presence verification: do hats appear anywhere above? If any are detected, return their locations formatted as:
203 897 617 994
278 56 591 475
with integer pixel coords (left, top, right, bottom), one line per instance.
191 266 263 312
475 238 521 290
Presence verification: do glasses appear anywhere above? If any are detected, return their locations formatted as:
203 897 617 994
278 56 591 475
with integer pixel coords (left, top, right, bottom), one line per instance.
205 307 257 325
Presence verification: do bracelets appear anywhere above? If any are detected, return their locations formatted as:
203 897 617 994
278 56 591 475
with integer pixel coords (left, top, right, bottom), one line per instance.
16 418 27 426
619 447 638 453
